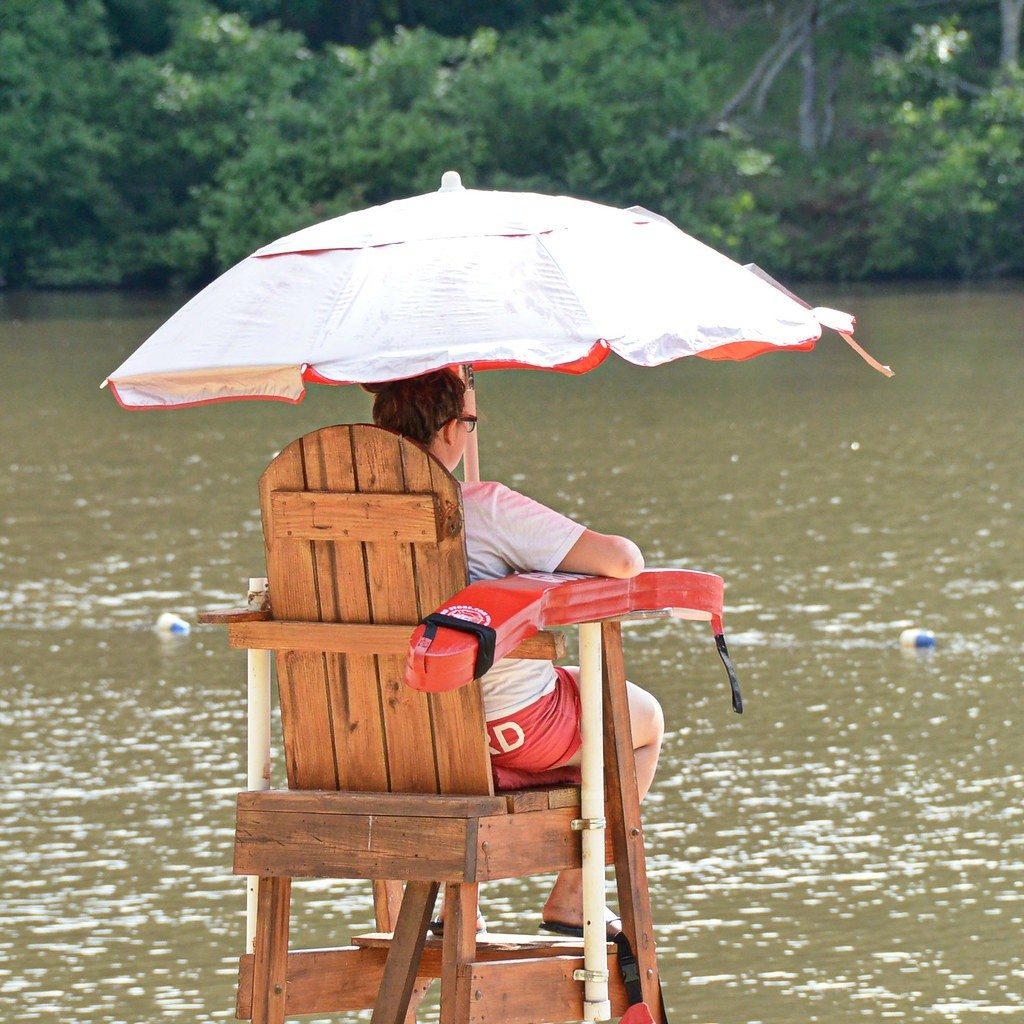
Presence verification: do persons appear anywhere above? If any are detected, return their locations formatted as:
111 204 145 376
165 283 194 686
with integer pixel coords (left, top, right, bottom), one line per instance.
360 366 664 941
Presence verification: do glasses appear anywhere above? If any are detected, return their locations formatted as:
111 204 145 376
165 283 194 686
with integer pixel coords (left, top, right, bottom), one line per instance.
436 414 478 433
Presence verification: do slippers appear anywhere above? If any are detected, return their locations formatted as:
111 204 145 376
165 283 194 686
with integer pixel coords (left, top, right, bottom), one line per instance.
539 917 622 942
430 912 487 935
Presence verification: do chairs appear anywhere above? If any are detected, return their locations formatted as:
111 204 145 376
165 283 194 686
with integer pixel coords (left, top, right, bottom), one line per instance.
196 424 740 1024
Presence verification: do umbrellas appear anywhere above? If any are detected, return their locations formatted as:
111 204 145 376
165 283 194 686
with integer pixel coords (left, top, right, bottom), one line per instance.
97 169 898 481
